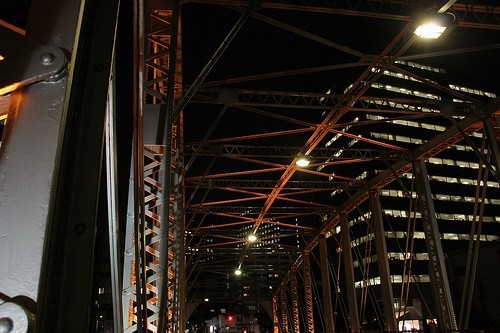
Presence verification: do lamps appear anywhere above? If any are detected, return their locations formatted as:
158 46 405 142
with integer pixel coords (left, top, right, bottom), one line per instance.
413 13 449 39
296 155 311 167
